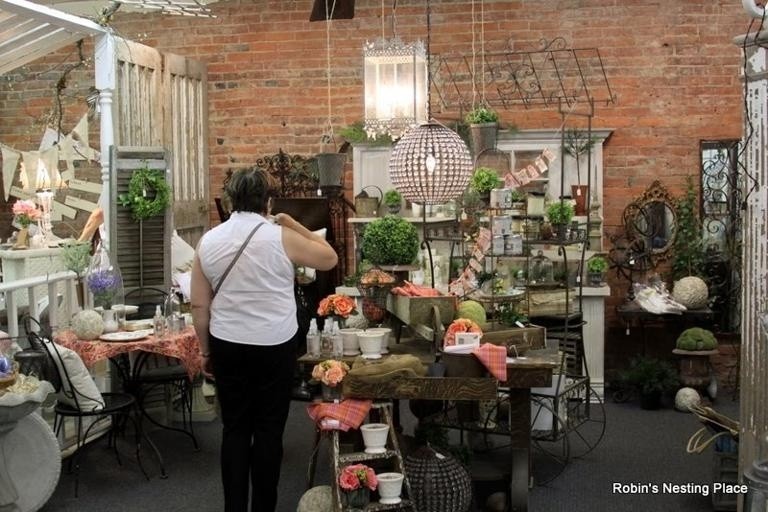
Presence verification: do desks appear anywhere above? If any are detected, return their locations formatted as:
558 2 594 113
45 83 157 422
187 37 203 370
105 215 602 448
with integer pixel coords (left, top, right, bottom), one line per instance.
615 302 716 401
296 333 563 512
1 245 93 314
53 315 204 484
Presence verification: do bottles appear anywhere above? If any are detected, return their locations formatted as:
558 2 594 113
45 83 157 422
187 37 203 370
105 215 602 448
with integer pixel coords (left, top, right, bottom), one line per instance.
153 304 167 337
490 182 526 256
306 316 345 360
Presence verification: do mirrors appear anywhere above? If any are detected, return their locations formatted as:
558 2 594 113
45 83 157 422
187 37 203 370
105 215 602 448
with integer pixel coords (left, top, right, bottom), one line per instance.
623 177 686 268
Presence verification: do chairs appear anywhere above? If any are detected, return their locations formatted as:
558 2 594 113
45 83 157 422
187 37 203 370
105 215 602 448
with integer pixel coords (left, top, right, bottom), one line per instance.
122 288 199 454
22 315 151 498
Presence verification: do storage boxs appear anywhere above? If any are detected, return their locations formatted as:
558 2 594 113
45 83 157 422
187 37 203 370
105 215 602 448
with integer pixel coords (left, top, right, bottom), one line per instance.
385 292 455 326
709 447 740 507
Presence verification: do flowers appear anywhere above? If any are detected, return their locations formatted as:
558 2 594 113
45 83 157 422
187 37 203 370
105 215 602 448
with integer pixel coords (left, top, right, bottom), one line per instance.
0 329 22 380
315 292 360 321
12 198 41 229
85 269 122 310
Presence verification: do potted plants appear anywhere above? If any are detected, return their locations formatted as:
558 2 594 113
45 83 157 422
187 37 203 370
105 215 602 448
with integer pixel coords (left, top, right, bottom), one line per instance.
611 354 679 412
337 108 610 288
669 325 720 383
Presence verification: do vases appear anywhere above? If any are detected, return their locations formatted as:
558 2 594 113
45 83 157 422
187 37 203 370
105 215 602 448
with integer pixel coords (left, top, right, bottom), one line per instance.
21 223 30 249
0 373 21 398
305 317 486 508
102 307 119 335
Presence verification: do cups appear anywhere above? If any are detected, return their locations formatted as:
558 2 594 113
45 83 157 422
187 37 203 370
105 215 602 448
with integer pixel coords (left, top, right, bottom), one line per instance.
407 201 448 284
341 327 393 359
375 470 406 504
359 421 390 456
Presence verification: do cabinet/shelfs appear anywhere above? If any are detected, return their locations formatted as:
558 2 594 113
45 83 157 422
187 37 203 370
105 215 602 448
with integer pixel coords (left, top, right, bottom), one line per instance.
420 95 608 488
214 147 350 395
517 290 610 407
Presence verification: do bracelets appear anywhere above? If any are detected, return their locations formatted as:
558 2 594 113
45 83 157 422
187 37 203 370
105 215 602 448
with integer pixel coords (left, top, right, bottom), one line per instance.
199 351 211 358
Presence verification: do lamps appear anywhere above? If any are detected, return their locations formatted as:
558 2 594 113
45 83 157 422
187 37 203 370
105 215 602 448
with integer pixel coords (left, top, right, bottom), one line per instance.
23 158 70 246
386 0 473 208
359 0 432 143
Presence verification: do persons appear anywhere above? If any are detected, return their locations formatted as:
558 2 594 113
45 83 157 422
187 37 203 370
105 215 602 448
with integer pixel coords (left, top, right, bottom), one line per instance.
190 167 339 512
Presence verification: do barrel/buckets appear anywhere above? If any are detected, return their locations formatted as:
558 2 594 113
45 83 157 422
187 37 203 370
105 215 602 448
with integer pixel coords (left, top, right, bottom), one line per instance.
315 135 348 188
470 100 499 155
355 184 383 218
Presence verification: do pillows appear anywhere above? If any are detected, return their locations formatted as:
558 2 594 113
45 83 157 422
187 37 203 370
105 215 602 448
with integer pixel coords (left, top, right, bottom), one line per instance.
170 230 195 288
36 335 105 414
122 316 181 376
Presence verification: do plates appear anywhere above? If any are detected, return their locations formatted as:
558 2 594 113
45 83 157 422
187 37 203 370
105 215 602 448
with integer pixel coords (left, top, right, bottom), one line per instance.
96 302 157 342
